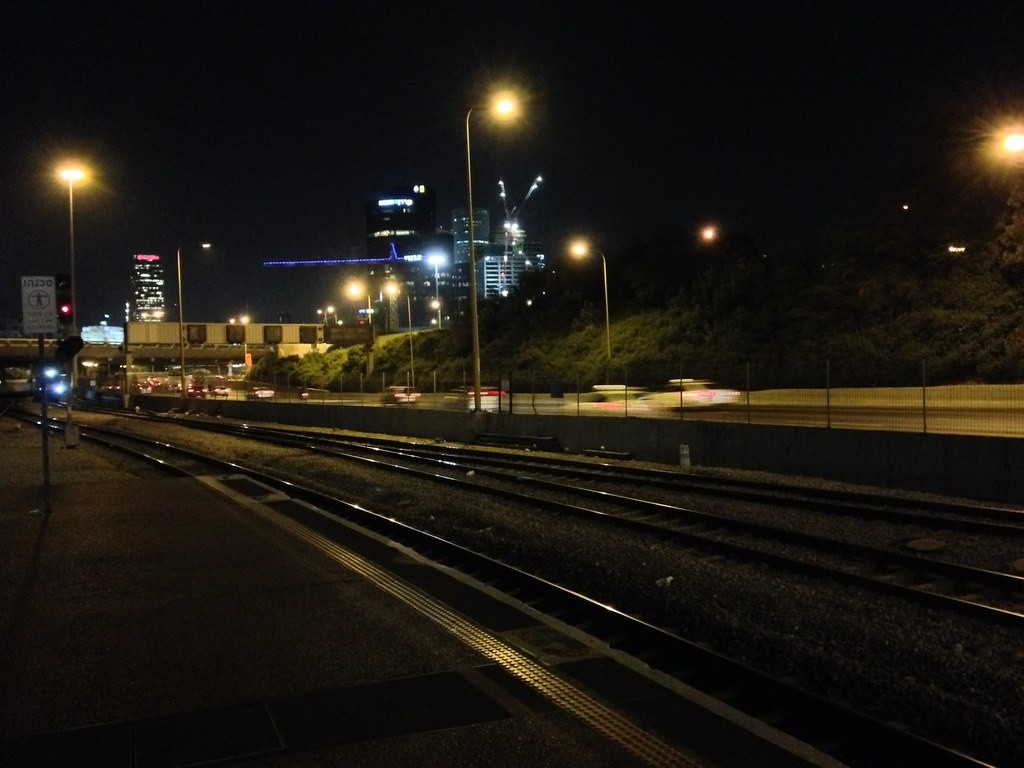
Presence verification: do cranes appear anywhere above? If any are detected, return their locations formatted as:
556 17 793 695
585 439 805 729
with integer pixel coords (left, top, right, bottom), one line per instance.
497 175 544 300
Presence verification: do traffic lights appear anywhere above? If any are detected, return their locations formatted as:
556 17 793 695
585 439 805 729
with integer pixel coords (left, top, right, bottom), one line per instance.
55 273 74 325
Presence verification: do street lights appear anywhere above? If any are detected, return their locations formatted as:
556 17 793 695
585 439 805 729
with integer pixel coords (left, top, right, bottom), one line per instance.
382 282 415 393
327 306 338 325
569 239 612 360
431 299 442 331
176 241 212 397
350 284 372 325
464 91 523 413
56 161 86 389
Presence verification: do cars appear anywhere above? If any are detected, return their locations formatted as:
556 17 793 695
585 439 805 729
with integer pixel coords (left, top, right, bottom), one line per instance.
132 363 331 403
380 385 422 405
443 385 507 413
